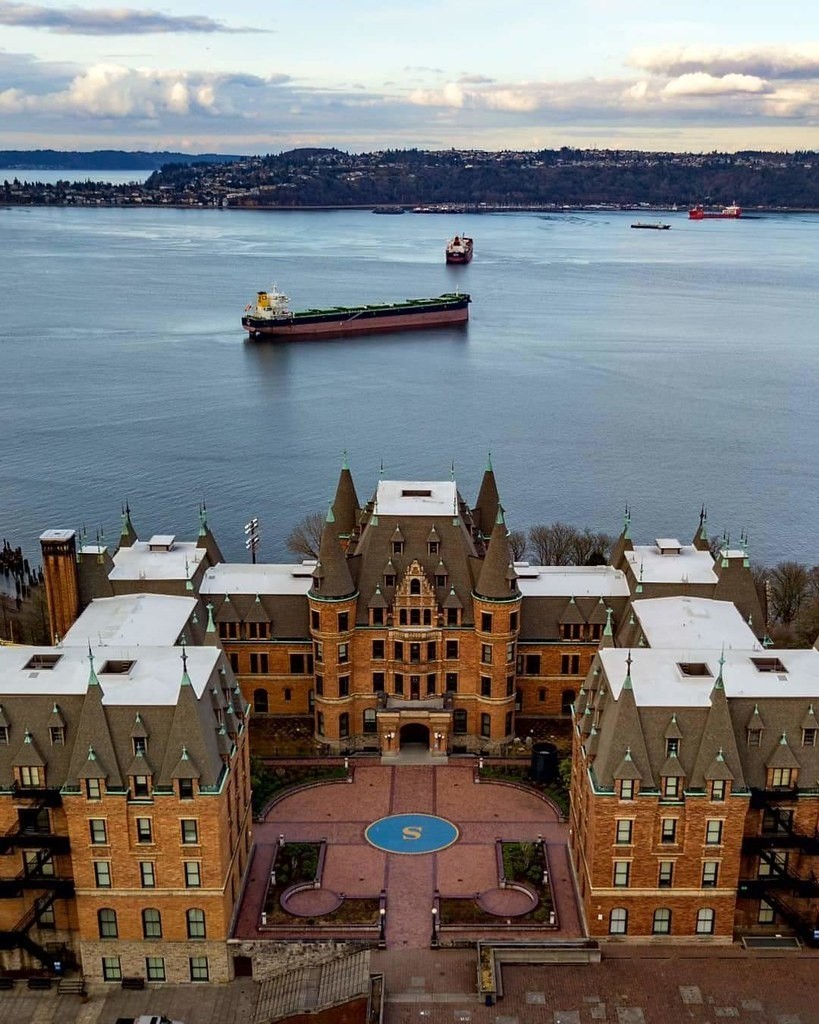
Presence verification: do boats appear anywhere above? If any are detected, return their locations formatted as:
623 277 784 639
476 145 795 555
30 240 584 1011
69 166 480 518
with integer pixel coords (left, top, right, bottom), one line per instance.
631 221 671 231
686 199 743 220
445 231 474 265
241 280 474 338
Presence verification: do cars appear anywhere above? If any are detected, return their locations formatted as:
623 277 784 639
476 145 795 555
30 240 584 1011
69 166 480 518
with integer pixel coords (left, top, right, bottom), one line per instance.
134 1014 183 1024
